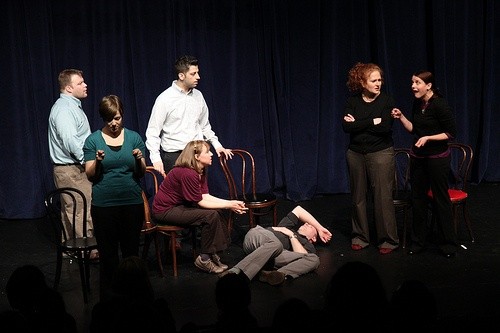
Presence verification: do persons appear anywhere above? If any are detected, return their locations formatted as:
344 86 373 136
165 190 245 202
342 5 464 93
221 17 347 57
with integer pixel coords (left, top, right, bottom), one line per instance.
0 256 439 333
47 69 100 260
145 55 250 274
342 62 403 255
83 95 147 305
391 70 459 259
216 205 333 286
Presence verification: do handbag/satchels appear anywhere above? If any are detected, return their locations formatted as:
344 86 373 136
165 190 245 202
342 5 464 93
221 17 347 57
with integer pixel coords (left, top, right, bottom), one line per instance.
409 147 452 170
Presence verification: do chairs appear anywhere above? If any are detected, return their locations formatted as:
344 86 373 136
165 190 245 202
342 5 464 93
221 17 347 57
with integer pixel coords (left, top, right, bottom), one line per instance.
42 186 100 305
219 147 279 232
139 165 187 278
427 142 476 242
392 144 411 250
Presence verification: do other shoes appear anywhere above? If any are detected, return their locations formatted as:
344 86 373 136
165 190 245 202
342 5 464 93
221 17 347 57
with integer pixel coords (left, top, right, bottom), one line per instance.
256 270 286 286
378 248 395 255
352 243 363 252
211 255 229 271
194 255 225 275
